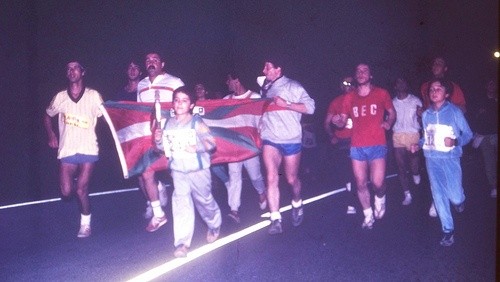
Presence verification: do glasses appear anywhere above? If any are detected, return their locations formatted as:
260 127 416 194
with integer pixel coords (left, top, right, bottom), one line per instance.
430 87 440 92
343 81 351 86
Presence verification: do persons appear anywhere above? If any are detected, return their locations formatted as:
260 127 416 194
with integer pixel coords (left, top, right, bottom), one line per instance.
223 71 267 224
45 59 104 237
116 49 229 232
259 56 315 234
324 53 500 247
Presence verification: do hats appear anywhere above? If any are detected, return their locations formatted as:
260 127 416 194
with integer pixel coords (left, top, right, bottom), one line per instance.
65 59 88 76
432 47 451 66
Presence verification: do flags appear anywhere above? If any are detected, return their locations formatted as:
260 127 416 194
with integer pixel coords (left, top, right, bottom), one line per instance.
100 99 267 179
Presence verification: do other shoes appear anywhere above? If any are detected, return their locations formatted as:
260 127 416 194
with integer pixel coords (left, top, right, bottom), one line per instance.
77 225 92 238
292 204 304 227
159 184 167 207
347 205 356 214
144 211 153 218
257 192 267 210
362 208 375 230
346 182 352 191
402 189 414 206
374 194 386 219
454 205 464 214
269 220 286 234
174 245 188 257
227 211 241 224
491 188 497 198
440 233 455 247
428 201 438 217
146 212 167 232
412 174 421 184
206 228 220 242
